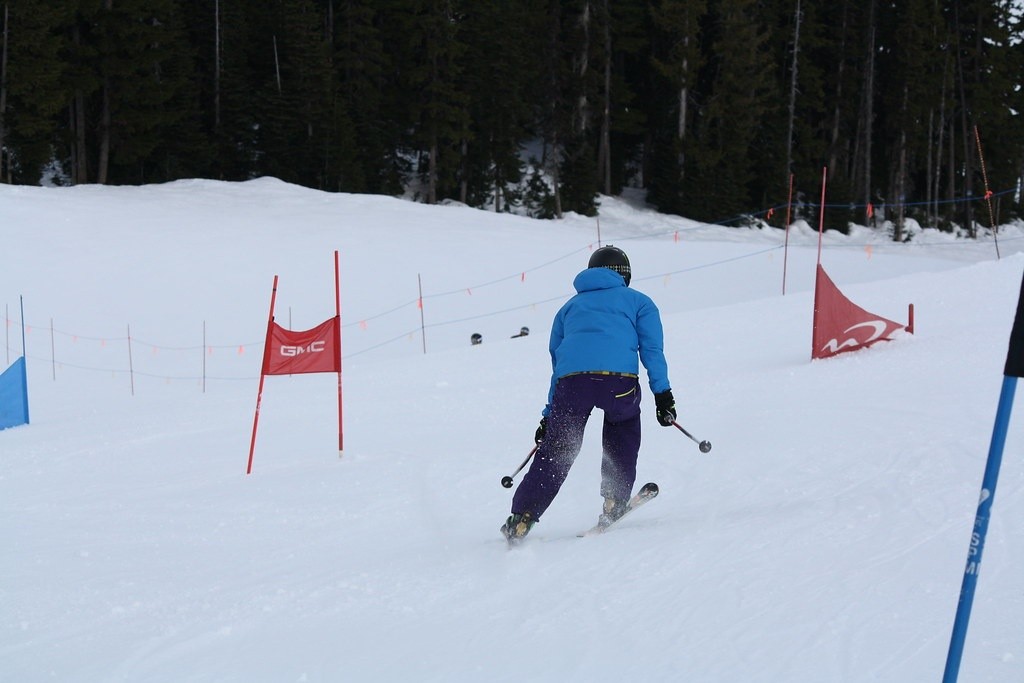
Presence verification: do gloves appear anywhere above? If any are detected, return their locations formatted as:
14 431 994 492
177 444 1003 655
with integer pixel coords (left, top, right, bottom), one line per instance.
654 389 676 427
535 417 548 445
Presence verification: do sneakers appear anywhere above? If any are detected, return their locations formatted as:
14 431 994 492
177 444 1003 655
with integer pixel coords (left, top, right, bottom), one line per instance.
597 498 626 526
500 509 539 548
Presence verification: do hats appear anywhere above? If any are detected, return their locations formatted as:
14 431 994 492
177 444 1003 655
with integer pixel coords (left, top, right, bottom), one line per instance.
588 244 631 279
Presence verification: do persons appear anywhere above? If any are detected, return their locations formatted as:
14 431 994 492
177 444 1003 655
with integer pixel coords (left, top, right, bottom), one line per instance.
500 245 677 545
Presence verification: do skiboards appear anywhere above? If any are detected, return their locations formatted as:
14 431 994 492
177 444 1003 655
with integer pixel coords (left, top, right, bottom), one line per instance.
573 482 658 538
500 517 523 555
